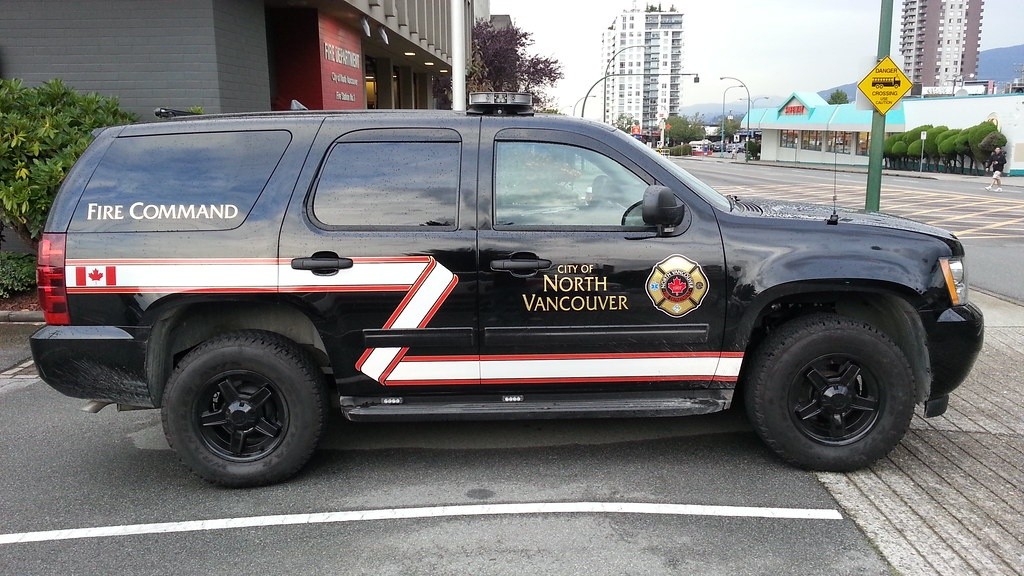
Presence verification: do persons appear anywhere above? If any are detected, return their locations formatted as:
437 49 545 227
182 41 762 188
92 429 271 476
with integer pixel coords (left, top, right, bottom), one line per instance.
731 148 736 160
985 147 1006 193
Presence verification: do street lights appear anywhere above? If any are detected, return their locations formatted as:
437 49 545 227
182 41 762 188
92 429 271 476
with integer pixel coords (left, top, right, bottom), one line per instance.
720 85 745 158
720 76 751 163
604 44 647 122
739 95 769 111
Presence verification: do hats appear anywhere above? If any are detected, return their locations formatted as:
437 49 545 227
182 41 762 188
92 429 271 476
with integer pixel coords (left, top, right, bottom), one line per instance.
546 162 581 182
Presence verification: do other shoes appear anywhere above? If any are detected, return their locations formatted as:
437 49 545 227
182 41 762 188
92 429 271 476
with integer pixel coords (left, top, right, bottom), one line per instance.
985 186 993 192
993 188 1002 192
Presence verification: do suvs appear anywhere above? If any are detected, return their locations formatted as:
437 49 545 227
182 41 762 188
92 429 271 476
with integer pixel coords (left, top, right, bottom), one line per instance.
28 91 986 490
